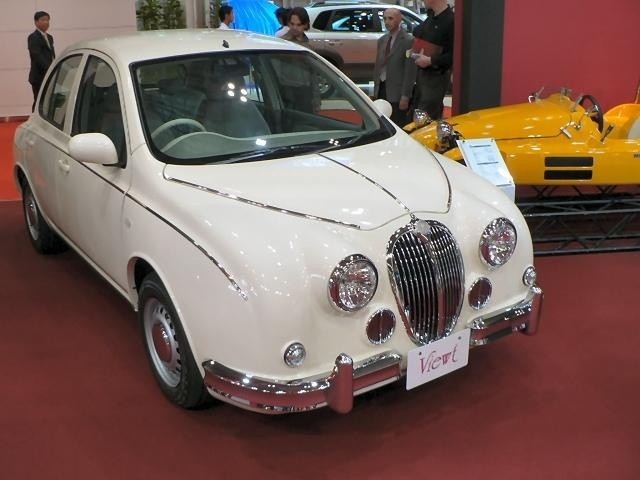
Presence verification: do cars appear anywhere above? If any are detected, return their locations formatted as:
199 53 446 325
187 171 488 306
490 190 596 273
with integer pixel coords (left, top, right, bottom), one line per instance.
274 0 453 98
13 28 544 416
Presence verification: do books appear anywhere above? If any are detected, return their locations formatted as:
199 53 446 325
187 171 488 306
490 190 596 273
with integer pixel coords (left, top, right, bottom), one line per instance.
410 37 444 63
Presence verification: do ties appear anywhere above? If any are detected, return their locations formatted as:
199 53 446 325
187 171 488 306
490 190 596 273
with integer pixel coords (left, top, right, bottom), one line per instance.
385 35 393 57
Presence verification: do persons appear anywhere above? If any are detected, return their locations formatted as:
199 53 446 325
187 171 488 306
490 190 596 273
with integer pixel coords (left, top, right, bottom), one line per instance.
373 8 417 130
274 6 292 39
25 11 57 116
218 4 238 29
279 6 323 115
405 0 455 123
